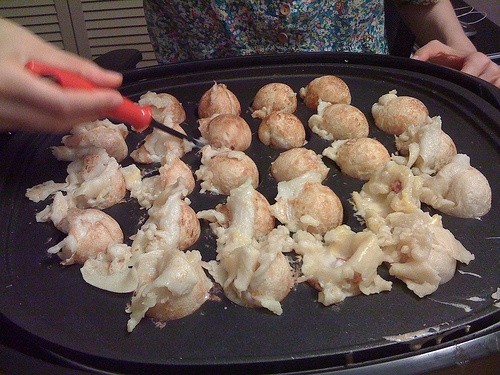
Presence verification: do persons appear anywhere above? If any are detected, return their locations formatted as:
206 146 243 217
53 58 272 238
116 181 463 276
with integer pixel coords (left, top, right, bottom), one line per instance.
0 0 500 137
